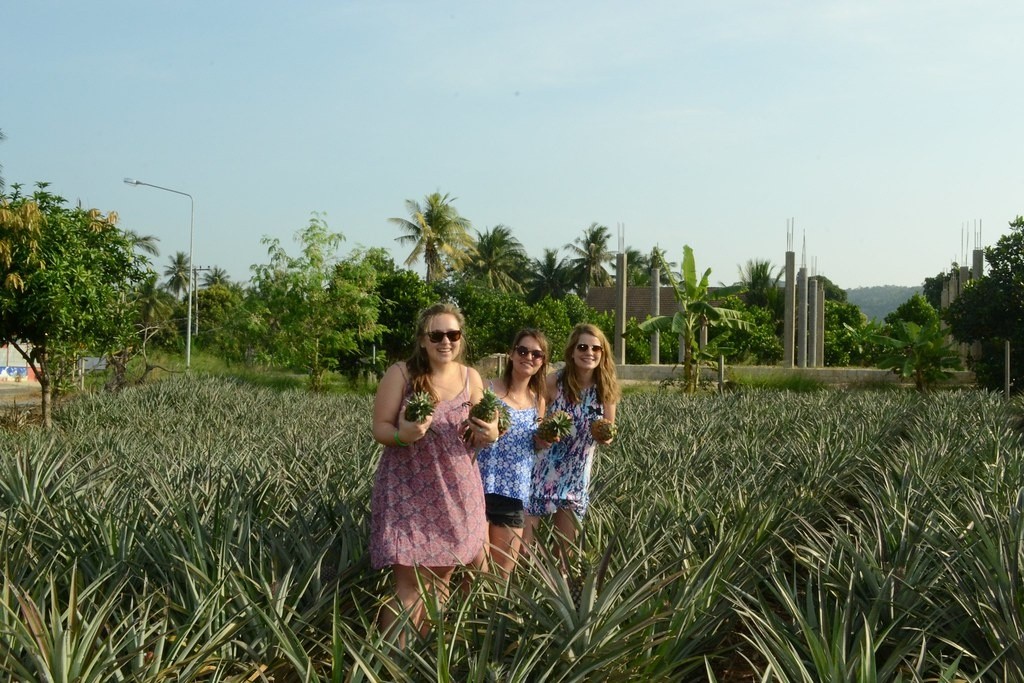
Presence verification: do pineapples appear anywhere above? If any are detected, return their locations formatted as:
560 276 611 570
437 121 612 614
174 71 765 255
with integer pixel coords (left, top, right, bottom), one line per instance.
590 419 614 441
537 411 574 442
469 389 511 435
404 392 433 422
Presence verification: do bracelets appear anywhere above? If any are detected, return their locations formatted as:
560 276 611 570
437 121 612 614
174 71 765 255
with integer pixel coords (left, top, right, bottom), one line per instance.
394 431 409 446
487 438 498 445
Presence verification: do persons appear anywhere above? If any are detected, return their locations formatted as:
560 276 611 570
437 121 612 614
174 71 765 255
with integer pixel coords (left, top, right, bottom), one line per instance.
368 303 499 648
519 325 619 557
462 330 560 601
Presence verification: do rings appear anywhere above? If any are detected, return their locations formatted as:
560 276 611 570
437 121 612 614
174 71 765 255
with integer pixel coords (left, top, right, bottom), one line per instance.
479 428 482 432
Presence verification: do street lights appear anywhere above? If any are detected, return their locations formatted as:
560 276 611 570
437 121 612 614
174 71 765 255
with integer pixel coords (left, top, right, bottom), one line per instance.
123 177 194 370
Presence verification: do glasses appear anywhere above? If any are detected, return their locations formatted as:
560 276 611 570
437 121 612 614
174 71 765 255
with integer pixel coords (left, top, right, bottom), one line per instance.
423 330 462 343
514 345 544 358
576 343 602 353
